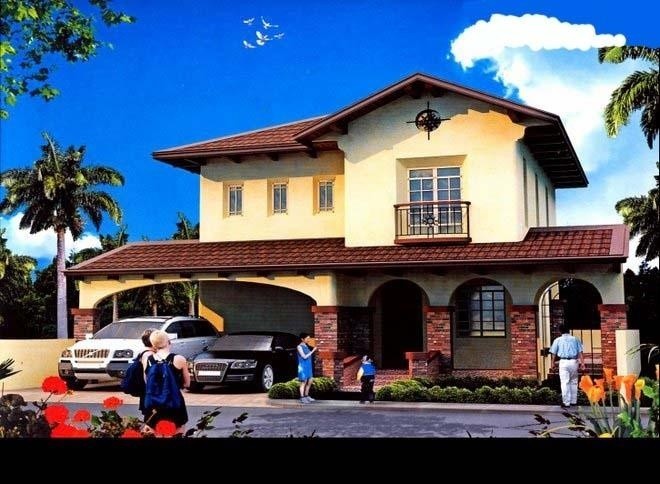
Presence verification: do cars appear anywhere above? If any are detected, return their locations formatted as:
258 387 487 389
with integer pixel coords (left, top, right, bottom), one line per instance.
187 329 305 389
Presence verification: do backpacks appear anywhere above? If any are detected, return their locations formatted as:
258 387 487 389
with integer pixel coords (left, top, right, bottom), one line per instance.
121 351 146 397
143 355 187 420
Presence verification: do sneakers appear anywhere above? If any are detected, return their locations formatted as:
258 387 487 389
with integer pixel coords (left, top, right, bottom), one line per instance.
300 396 315 404
561 404 576 408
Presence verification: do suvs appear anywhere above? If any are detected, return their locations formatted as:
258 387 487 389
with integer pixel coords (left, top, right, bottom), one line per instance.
59 313 218 392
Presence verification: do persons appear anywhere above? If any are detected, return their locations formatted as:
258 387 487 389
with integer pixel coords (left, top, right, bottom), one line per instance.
357 354 376 404
138 329 158 429
296 332 317 404
143 330 192 438
548 323 585 408
